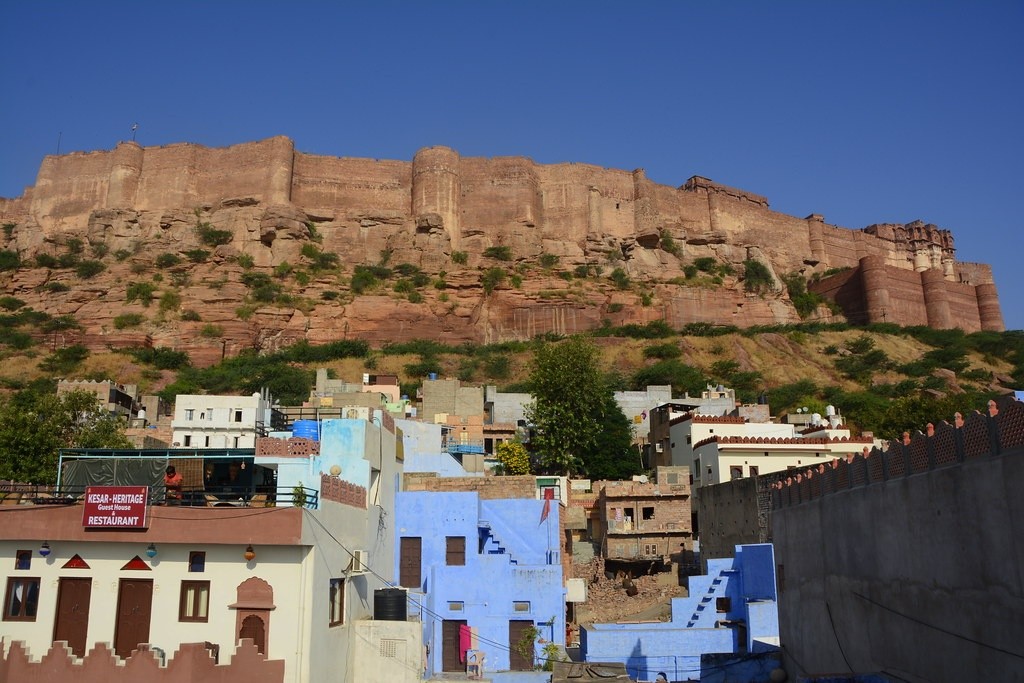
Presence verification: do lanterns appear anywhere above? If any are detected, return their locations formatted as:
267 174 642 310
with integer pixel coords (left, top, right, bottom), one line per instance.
39 543 51 557
146 545 158 558
244 547 256 563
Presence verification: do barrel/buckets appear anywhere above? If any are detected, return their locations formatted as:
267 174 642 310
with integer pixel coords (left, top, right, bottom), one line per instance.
373 589 406 621
429 373 437 380
292 419 321 441
811 405 842 429
402 394 408 400
138 410 145 418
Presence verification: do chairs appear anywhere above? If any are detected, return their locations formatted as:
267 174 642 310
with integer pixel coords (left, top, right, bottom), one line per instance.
249 495 268 508
466 651 488 678
0 492 24 505
205 495 220 507
33 492 56 502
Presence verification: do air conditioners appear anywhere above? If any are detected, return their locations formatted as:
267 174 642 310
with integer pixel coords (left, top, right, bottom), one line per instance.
353 550 368 572
342 407 374 423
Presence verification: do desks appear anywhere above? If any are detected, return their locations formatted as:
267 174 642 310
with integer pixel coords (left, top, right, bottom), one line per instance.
209 500 251 507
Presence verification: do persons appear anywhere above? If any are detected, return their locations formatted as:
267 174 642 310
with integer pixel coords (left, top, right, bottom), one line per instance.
164 466 183 506
565 624 574 646
147 422 151 428
640 410 646 420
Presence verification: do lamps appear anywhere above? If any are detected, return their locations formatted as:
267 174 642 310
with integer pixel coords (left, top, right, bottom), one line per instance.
146 543 157 558
39 540 50 556
241 458 245 469
245 545 255 561
